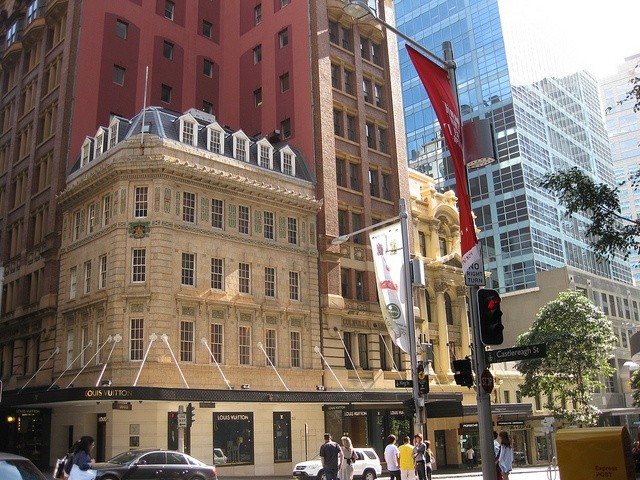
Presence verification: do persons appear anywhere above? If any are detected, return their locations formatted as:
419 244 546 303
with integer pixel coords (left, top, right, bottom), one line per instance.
493 429 504 480
319 432 344 480
60 434 115 480
339 436 357 480
383 434 401 480
422 439 433 480
465 445 475 469
397 435 416 480
411 432 429 480
496 430 516 480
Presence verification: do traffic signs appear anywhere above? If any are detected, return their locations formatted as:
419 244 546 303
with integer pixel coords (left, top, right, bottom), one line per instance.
486 343 547 364
178 413 187 429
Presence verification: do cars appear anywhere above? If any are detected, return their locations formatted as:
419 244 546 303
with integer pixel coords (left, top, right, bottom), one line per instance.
84 447 222 479
213 447 228 465
1 451 48 480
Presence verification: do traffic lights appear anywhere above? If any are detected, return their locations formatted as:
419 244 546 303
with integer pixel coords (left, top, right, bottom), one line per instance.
453 359 470 386
191 406 195 424
481 294 505 338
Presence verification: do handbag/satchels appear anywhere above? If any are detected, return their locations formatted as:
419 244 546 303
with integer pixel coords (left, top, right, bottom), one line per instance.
352 451 358 463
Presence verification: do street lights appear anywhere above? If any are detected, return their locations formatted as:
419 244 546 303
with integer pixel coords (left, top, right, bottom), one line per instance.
329 197 427 439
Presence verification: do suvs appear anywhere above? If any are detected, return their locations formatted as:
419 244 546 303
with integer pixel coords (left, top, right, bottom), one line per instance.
292 448 383 480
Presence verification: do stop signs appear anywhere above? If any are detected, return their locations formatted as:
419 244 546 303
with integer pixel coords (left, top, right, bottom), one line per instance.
481 370 493 394
